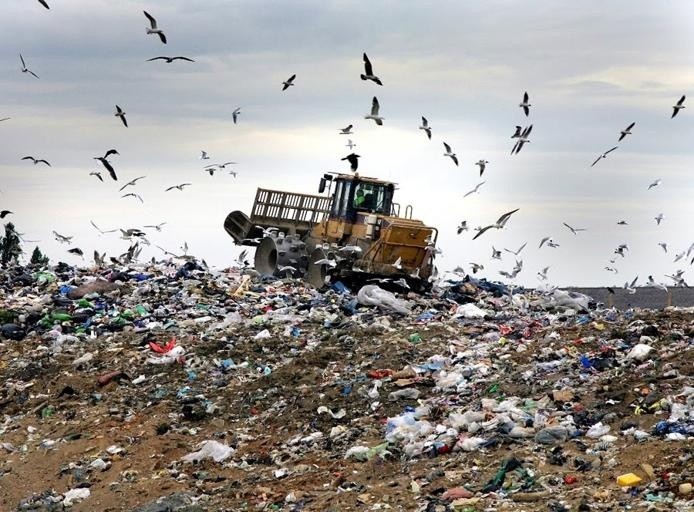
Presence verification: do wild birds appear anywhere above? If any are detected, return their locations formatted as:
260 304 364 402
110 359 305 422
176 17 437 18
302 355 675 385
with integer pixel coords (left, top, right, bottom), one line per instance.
590 122 636 167
282 74 297 91
143 10 194 64
232 108 241 125
508 91 534 156
454 208 693 294
671 95 686 119
0 208 196 282
88 149 238 204
418 115 459 167
648 178 662 189
21 156 50 167
462 160 490 198
19 53 39 79
233 225 443 289
115 104 128 128
337 52 386 173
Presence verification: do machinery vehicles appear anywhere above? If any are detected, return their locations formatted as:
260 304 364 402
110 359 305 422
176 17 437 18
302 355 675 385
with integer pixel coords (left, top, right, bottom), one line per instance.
224 171 443 294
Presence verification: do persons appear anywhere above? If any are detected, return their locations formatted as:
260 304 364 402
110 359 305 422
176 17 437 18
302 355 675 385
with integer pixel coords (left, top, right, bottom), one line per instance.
354 189 366 207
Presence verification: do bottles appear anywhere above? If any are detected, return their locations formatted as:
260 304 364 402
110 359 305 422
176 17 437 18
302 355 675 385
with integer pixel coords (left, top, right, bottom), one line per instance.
662 472 682 483
